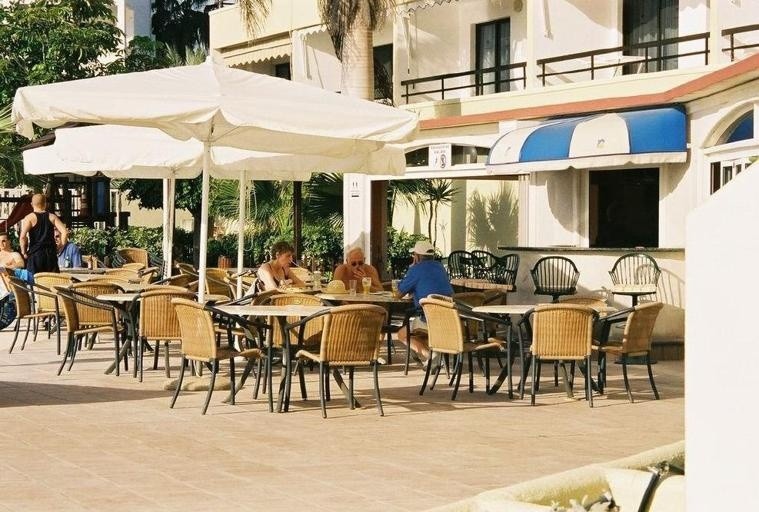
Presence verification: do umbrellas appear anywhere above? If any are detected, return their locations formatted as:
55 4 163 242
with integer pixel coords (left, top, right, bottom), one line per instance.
11 54 420 304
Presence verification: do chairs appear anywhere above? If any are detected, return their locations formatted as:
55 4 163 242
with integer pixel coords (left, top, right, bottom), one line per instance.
448 250 486 279
217 288 279 382
183 279 199 291
117 262 144 284
224 277 251 301
404 296 455 376
472 250 500 274
113 261 161 279
289 266 319 288
85 274 133 287
474 254 520 292
428 294 507 390
137 267 158 287
283 303 384 418
129 291 198 381
529 256 579 303
1 268 35 331
50 286 128 377
91 268 140 290
225 270 235 277
150 273 189 289
555 297 606 389
205 267 231 297
177 263 201 291
419 298 514 399
608 253 660 364
594 301 659 402
250 294 333 401
138 263 161 285
113 267 162 278
169 298 276 414
118 247 163 281
6 274 67 356
34 272 75 344
517 304 600 408
74 259 103 277
71 256 109 268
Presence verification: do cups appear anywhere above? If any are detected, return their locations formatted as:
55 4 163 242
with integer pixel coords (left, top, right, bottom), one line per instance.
349 279 357 296
362 277 371 294
392 280 399 297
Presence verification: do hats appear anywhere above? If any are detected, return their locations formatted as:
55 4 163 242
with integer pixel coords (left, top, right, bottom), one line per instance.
409 240 435 256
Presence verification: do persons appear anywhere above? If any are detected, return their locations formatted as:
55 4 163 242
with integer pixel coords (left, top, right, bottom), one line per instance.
1 232 24 299
20 194 68 330
52 227 81 268
257 241 305 290
334 247 387 365
397 241 455 371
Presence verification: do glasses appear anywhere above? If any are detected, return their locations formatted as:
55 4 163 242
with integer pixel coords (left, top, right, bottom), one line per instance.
349 261 364 266
55 235 59 238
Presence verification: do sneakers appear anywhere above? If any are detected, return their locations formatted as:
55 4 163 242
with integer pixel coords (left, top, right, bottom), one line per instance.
423 352 440 372
